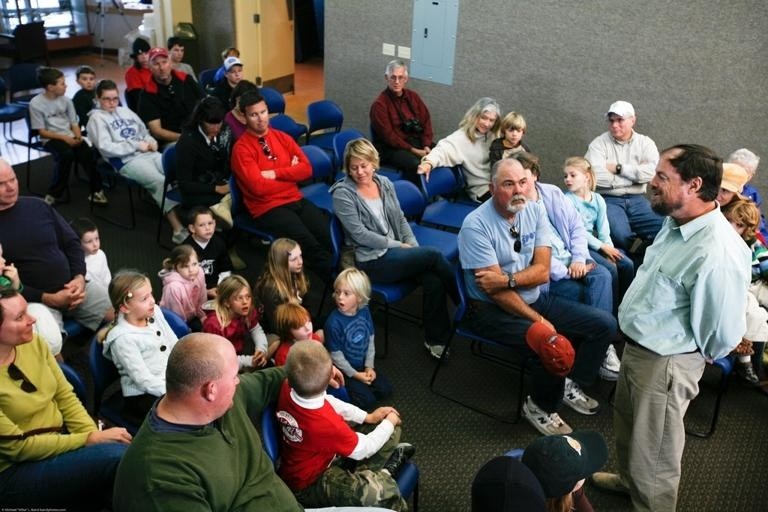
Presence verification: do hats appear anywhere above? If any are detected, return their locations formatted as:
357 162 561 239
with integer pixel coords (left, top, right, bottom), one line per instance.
522 430 608 498
223 56 243 72
148 48 168 60
130 37 150 57
471 455 548 512
605 101 635 118
526 321 576 376
720 162 748 193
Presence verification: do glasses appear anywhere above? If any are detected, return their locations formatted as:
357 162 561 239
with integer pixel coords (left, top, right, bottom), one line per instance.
607 118 624 123
510 225 522 253
259 136 271 155
8 363 37 392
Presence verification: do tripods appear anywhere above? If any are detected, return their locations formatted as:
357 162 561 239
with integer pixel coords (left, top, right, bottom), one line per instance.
87 2 134 65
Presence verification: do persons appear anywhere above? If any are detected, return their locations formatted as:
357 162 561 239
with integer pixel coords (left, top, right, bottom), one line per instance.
4 38 768 511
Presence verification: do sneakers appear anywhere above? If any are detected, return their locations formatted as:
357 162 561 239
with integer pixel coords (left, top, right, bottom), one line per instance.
88 190 108 203
522 396 573 436
425 340 451 359
599 344 622 380
564 377 600 415
593 472 630 493
736 363 759 383
385 442 415 480
226 249 247 270
172 228 190 243
